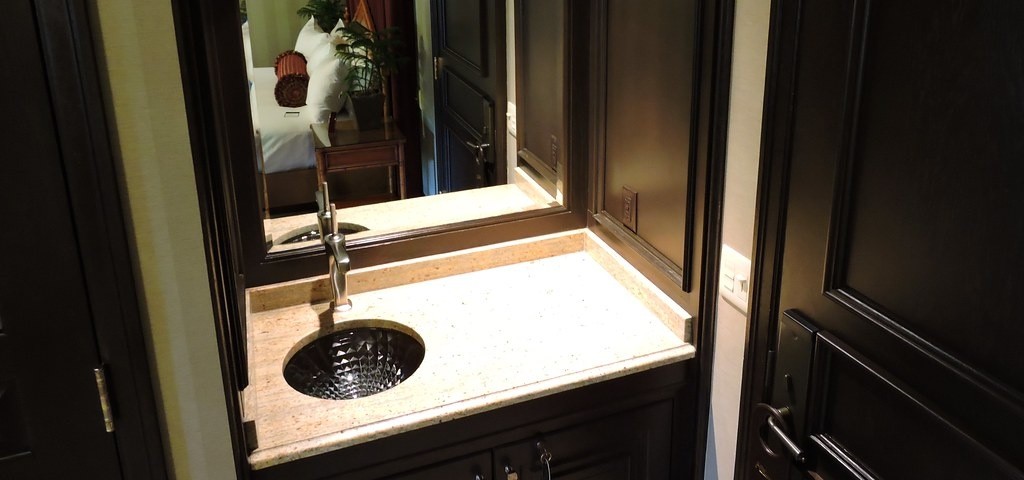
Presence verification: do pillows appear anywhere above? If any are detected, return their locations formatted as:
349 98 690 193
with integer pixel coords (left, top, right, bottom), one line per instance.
273 51 310 110
292 14 330 58
303 59 352 123
306 42 339 75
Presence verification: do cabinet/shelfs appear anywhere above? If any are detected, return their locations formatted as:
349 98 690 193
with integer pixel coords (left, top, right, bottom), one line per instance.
238 228 699 480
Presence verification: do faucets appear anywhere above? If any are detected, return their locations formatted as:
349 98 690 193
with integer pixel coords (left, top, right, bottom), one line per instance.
316 183 339 242
325 203 354 309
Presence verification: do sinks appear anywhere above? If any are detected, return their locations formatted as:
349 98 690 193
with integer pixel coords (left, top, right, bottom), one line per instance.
282 318 426 400
273 223 368 245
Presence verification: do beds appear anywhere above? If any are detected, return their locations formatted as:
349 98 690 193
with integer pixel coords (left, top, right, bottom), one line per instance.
249 0 391 211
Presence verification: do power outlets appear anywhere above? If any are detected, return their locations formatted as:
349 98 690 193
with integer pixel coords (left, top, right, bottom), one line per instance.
621 184 639 233
550 134 559 171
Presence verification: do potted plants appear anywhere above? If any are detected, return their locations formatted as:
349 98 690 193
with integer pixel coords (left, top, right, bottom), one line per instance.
335 21 408 131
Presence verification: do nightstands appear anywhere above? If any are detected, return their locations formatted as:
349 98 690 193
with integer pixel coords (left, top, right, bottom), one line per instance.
309 119 408 199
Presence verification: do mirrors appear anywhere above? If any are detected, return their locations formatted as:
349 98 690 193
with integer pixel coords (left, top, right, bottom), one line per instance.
197 0 587 284
